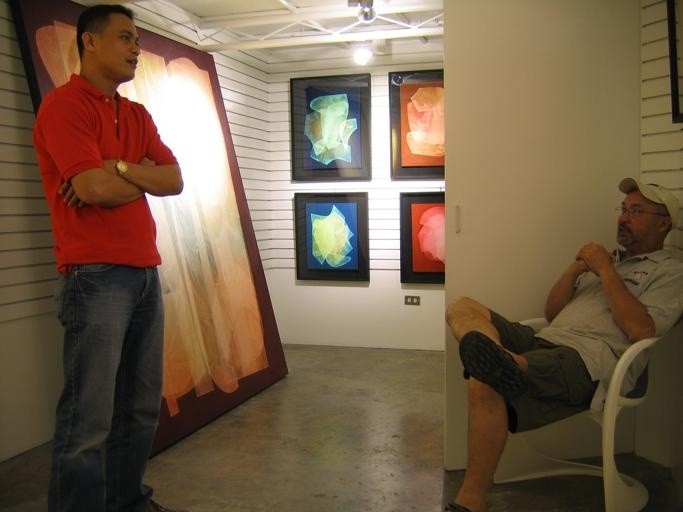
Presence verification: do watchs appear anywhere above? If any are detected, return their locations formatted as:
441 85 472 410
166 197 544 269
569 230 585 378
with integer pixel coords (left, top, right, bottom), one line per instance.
114 157 129 178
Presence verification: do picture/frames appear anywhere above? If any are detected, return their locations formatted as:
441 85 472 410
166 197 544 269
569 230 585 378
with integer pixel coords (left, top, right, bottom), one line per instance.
294 192 370 281
399 192 445 283
388 69 445 181
290 73 372 180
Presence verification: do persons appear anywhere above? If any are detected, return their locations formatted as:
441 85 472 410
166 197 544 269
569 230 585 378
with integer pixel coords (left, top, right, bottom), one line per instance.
31 3 183 512
440 173 683 512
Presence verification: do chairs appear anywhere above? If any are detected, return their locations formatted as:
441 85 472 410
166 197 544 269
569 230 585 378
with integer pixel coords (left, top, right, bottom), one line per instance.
520 317 662 511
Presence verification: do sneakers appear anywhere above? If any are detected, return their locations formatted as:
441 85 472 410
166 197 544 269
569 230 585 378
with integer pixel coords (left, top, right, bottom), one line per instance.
458 330 527 398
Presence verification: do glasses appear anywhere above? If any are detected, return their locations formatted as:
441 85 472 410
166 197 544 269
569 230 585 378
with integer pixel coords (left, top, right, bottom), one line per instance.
615 207 667 218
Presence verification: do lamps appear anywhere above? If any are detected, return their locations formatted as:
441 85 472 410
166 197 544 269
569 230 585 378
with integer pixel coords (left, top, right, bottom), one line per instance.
346 1 377 23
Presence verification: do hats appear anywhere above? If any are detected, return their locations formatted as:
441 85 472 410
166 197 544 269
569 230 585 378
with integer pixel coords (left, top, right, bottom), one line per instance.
618 177 680 230
